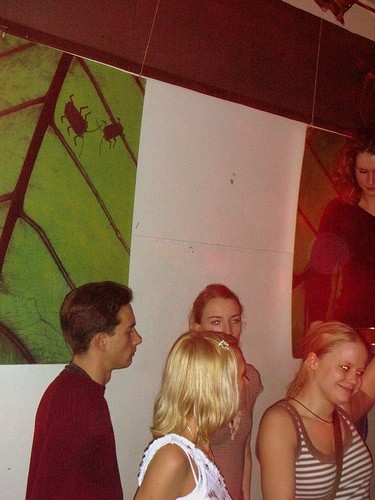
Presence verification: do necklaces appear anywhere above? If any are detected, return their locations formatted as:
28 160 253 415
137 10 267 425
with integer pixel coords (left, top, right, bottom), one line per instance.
291 398 334 424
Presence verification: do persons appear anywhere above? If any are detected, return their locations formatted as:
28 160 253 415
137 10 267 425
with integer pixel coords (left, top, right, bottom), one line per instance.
256 320 375 500
134 330 250 500
303 139 375 356
24 280 142 500
187 283 264 500
